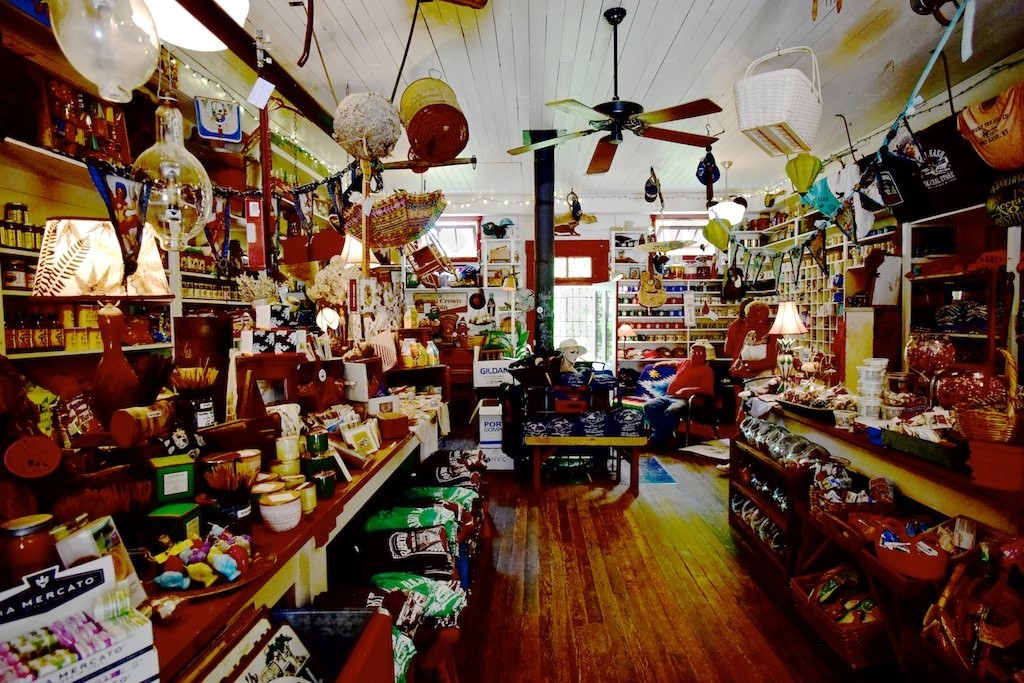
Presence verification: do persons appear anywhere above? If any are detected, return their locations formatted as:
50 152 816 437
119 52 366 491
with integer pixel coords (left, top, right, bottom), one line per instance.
716 300 783 471
556 339 587 372
724 298 754 433
415 299 425 313
643 345 715 449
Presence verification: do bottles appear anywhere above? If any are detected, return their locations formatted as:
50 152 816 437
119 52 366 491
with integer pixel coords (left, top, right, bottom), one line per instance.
3 304 65 354
639 234 645 245
739 417 853 490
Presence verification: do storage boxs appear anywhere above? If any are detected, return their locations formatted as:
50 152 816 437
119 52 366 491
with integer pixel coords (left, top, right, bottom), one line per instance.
375 410 410 440
143 502 205 543
57 302 105 352
471 358 519 388
857 397 883 419
862 357 890 377
468 396 505 449
858 387 883 397
857 379 884 389
0 554 162 683
833 409 858 429
854 365 885 381
174 604 397 683
148 452 196 502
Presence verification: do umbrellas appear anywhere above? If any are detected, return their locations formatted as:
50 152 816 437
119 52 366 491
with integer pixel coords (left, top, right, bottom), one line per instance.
344 189 447 257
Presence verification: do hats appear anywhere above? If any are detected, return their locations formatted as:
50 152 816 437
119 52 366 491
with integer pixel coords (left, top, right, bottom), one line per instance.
691 343 706 348
555 339 587 355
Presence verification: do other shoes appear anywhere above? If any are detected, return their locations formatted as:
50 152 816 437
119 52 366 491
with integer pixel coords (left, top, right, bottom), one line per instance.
662 439 678 452
642 437 656 451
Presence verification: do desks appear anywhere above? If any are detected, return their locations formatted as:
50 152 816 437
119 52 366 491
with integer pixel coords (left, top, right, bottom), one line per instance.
524 433 651 495
474 443 515 472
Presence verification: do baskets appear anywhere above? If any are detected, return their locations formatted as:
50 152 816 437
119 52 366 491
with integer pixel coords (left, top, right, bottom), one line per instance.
734 46 824 157
956 347 1024 444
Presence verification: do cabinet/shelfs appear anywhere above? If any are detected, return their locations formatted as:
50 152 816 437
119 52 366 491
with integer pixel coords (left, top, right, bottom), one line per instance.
0 1 498 683
603 204 1024 683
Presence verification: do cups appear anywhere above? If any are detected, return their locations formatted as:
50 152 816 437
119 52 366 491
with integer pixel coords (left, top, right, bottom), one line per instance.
194 429 336 532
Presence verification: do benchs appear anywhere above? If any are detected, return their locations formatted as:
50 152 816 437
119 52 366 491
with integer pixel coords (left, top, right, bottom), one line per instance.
614 362 727 449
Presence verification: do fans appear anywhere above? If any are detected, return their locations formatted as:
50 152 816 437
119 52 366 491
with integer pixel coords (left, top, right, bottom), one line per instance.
506 4 725 177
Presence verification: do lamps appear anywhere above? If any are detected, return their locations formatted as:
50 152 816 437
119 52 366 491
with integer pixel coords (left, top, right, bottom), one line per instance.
768 299 810 394
605 120 626 146
616 321 638 360
